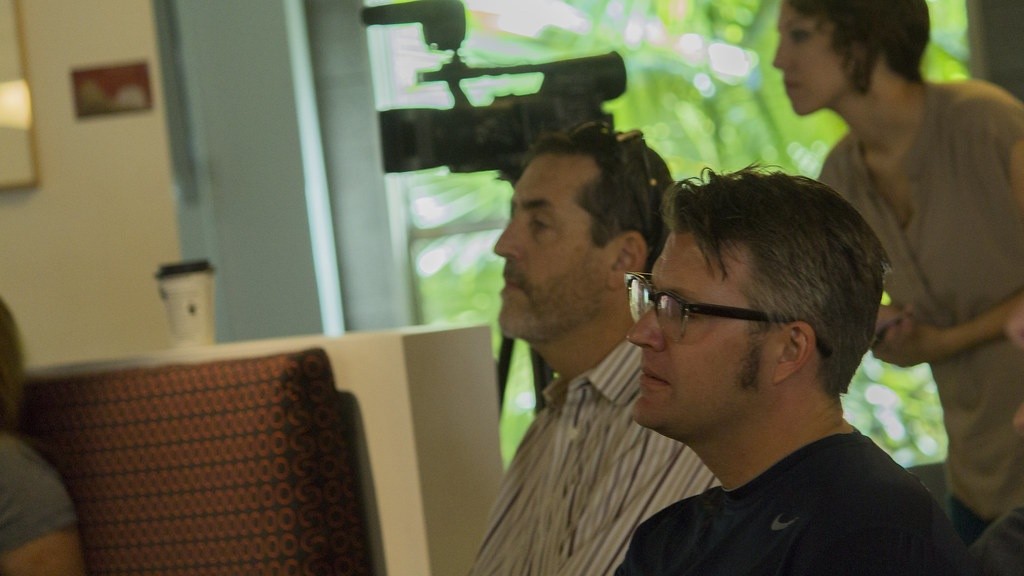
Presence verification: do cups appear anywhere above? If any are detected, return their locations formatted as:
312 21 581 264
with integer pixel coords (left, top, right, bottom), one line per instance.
154 257 217 347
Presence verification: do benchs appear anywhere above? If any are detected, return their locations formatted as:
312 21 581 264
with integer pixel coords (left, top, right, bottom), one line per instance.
0 348 372 576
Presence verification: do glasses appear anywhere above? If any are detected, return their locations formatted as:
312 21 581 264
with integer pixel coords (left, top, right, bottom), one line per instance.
620 131 662 241
622 270 832 358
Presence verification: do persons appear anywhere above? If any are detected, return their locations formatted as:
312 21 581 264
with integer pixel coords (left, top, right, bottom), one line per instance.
465 119 1024 576
772 1 1024 543
1 306 83 576
